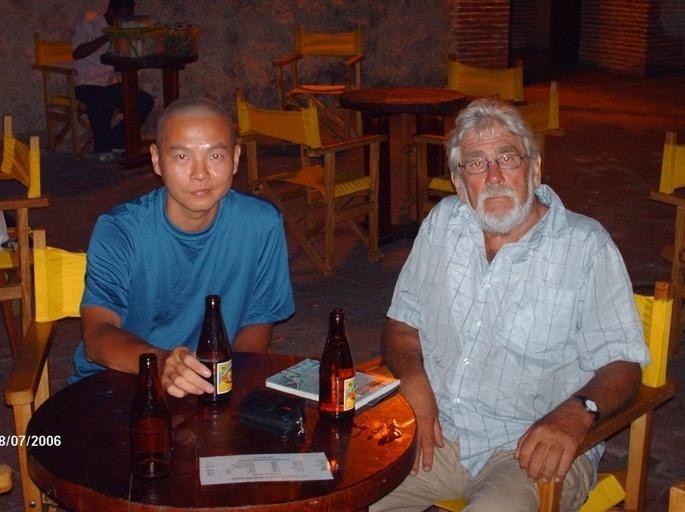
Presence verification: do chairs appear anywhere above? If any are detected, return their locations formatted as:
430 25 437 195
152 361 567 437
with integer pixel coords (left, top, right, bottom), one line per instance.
649 132 684 366
426 280 684 510
0 23 561 276
6 229 90 511
1 110 49 359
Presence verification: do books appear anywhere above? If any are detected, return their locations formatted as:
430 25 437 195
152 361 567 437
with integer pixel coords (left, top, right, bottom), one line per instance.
265 358 402 414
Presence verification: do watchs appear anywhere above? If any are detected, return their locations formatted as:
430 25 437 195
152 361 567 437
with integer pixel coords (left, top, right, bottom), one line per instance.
568 394 601 426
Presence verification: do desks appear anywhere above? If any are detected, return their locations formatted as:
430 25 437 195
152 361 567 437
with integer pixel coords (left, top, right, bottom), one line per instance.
25 349 417 511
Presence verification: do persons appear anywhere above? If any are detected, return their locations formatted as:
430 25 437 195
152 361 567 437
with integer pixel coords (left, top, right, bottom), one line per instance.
367 99 651 511
65 96 296 400
72 1 155 165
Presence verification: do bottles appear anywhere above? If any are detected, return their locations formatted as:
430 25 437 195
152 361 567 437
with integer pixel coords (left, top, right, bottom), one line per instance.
129 347 172 481
193 294 233 407
318 308 356 424
301 420 353 498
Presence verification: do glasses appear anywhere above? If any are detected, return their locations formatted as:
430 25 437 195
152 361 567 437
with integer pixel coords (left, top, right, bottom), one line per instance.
459 153 526 175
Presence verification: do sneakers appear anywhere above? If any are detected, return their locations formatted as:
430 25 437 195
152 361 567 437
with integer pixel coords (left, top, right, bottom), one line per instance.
96 148 127 162
